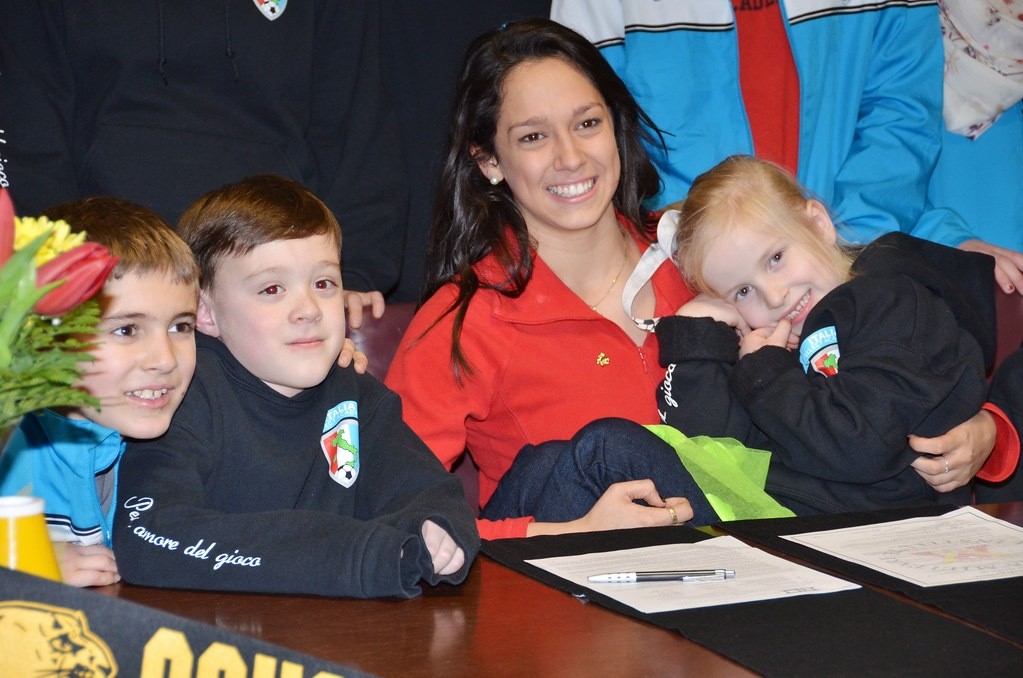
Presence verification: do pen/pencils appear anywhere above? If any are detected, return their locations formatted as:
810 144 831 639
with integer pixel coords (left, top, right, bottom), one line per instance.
589 568 737 584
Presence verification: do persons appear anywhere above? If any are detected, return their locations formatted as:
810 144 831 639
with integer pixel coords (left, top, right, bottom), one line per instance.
376 19 1021 545
1 1 422 325
105 169 480 597
549 1 949 247
917 90 1023 299
478 152 992 524
0 191 203 593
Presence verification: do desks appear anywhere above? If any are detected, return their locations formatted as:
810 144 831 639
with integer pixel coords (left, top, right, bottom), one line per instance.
98 497 1023 678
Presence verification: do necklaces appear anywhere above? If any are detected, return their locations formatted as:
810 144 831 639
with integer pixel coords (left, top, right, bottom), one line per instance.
586 227 627 315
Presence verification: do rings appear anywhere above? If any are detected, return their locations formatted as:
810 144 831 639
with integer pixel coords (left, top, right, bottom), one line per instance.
662 505 680 529
938 452 948 474
